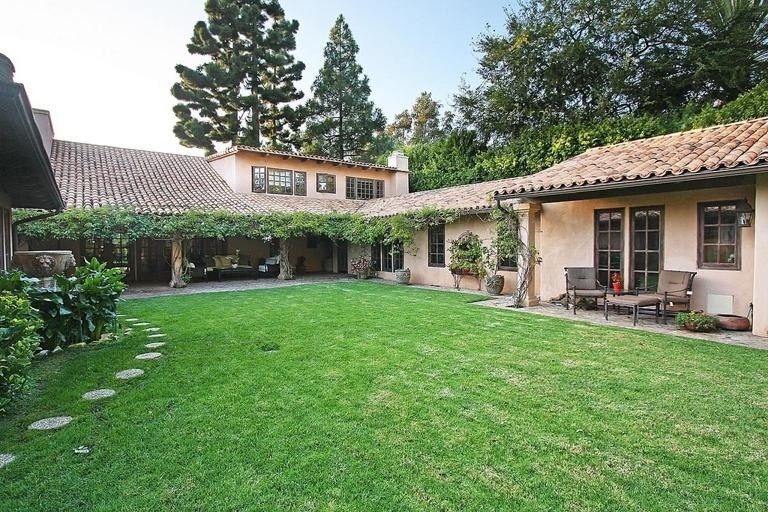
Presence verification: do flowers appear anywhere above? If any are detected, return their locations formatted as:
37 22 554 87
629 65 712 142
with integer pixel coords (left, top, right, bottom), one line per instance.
610 272 621 283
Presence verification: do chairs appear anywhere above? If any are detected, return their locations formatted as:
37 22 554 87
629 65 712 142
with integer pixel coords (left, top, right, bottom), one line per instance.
564 267 606 315
634 269 697 324
187 257 208 283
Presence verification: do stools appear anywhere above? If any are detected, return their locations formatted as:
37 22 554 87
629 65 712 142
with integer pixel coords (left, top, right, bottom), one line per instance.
604 296 660 325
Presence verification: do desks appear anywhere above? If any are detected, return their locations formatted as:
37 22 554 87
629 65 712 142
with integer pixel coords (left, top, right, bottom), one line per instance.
212 268 258 282
601 288 634 309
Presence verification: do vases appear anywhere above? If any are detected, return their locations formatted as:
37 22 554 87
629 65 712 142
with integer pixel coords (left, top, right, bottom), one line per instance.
612 282 622 291
714 314 750 331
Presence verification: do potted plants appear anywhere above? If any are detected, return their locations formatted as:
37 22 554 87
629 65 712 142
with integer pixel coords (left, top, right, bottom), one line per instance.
676 308 718 332
445 232 482 279
388 239 419 284
350 254 376 279
480 245 504 295
225 249 240 268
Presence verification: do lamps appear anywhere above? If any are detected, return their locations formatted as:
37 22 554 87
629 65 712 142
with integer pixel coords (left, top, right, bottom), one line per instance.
735 199 755 228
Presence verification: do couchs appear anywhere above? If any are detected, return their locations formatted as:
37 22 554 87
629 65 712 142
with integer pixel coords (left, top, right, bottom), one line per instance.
202 254 254 281
